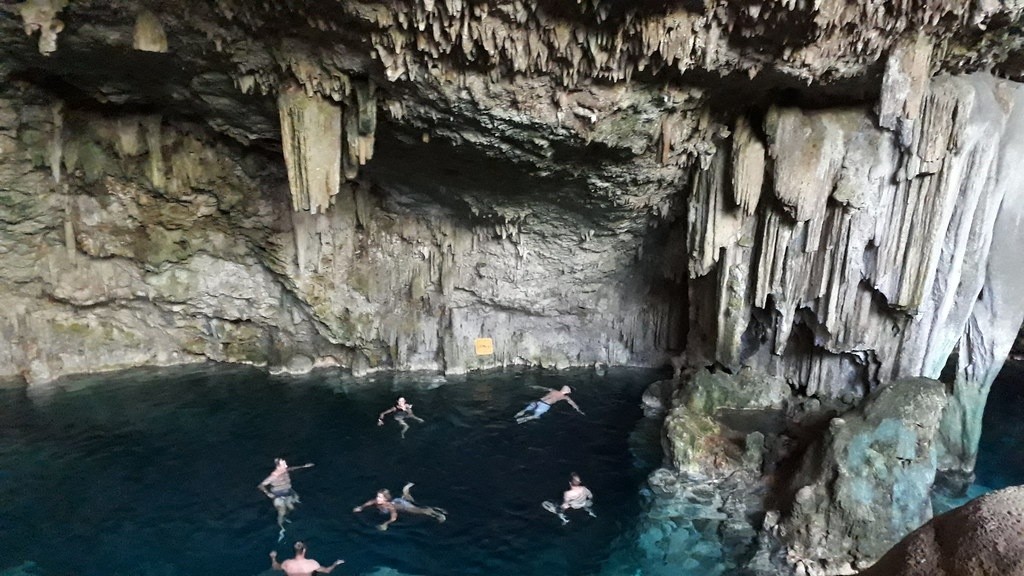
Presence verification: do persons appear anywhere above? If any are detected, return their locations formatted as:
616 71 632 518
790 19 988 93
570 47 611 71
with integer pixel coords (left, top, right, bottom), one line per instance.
270 541 346 576
257 458 314 528
557 474 597 524
515 384 586 424
378 396 423 438
353 482 449 533
